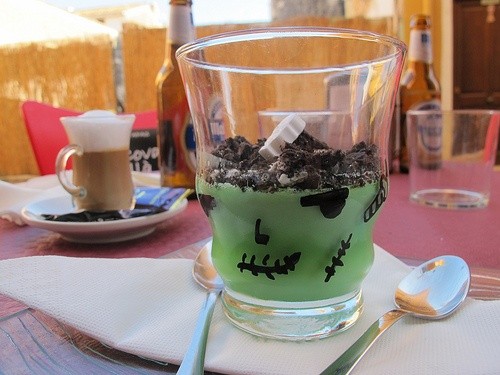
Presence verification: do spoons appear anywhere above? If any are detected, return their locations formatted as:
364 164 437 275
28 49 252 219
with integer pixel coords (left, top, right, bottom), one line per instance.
176 241 226 375
319 255 471 375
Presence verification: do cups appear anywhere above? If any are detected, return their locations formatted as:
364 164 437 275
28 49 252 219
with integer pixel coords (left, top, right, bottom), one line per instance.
406 110 500 209
55 113 136 213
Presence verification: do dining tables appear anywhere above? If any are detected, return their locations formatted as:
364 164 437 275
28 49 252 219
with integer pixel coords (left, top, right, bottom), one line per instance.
0 150 499 375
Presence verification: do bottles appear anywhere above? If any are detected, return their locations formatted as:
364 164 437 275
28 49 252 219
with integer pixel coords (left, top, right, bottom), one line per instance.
396 12 442 172
154 0 216 199
175 25 407 341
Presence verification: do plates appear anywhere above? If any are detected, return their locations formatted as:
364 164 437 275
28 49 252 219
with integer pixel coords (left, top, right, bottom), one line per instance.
20 188 189 244
